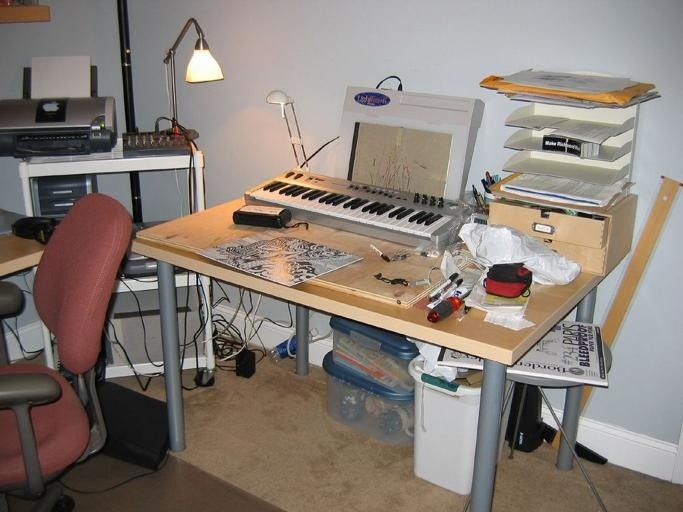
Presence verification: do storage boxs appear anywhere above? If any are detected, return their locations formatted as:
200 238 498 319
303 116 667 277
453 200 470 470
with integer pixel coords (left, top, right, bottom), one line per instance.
326 316 418 393
488 189 641 277
323 349 413 447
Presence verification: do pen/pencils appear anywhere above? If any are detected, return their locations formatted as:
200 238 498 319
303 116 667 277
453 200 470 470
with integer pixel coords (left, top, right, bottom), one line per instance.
472 185 482 209
481 172 503 194
437 279 464 301
428 273 459 297
370 244 391 262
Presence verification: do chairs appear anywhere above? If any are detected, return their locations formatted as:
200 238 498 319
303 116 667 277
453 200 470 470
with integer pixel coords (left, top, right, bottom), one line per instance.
0 194 132 511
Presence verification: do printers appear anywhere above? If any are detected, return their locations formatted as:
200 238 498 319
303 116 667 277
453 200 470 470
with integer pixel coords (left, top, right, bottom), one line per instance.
1 66 117 159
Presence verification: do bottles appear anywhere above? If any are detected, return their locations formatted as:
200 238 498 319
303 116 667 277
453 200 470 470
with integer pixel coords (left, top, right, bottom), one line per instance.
271 328 317 364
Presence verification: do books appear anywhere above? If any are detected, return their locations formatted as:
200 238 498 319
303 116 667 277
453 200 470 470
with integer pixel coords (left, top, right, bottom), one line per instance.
468 265 537 320
436 320 609 389
500 173 632 212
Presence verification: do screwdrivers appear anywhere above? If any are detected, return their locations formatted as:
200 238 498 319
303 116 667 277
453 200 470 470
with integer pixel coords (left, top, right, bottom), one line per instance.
427 290 472 322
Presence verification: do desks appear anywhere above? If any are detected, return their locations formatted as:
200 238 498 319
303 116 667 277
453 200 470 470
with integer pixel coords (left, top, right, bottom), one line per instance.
127 174 634 511
0 233 48 371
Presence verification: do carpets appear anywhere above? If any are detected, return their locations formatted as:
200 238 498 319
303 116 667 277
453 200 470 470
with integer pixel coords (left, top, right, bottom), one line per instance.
0 451 287 512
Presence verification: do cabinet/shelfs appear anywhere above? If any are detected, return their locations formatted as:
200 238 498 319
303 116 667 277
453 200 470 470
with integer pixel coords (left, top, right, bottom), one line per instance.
18 137 216 390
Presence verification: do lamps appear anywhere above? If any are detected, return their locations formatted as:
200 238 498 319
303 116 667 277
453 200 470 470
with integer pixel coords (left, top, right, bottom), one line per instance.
163 17 224 137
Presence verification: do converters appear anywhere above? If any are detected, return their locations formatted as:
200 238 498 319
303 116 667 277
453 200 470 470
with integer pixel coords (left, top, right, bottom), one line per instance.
235 350 254 377
